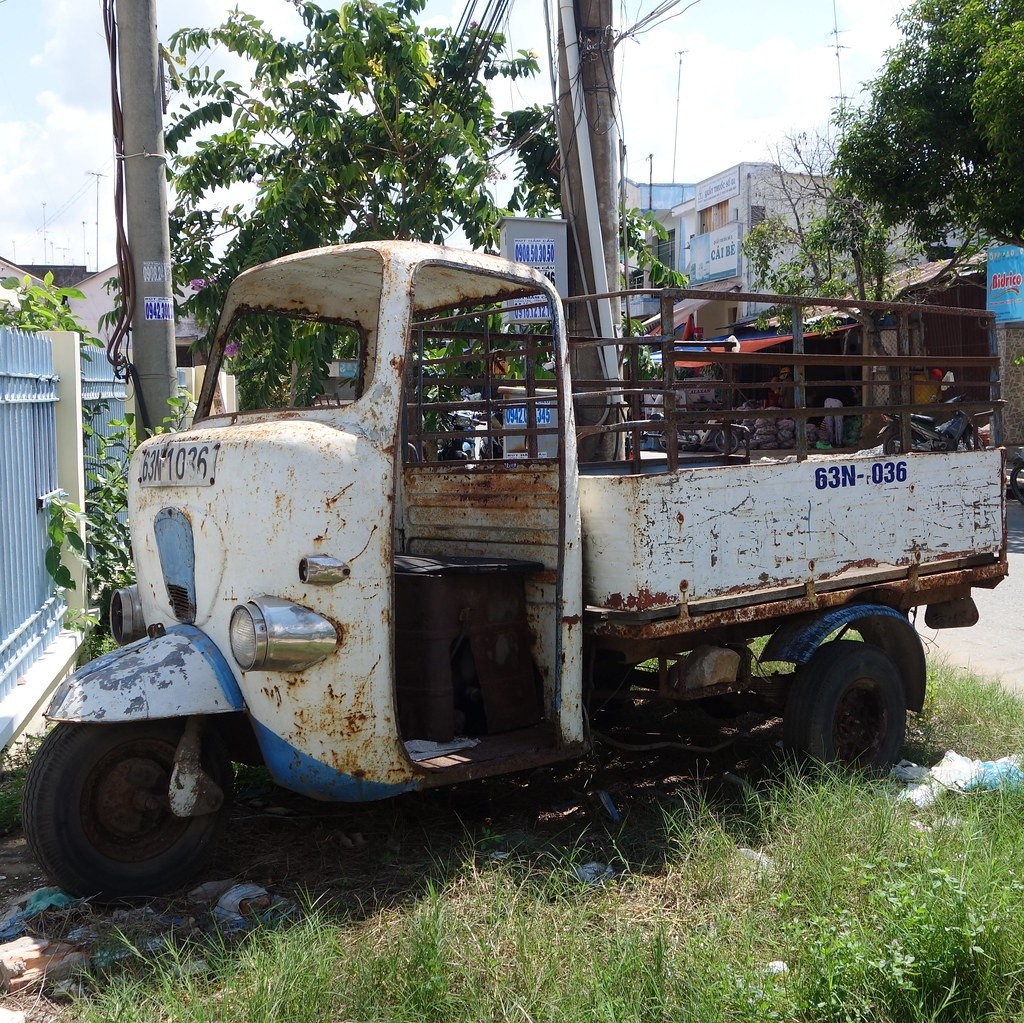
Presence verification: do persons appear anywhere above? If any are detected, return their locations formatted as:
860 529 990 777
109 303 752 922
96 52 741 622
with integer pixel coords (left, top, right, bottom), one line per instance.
768 373 793 408
824 393 850 447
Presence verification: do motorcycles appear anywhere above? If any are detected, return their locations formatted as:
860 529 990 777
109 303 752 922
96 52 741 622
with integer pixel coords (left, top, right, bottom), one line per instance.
876 391 985 456
658 399 740 454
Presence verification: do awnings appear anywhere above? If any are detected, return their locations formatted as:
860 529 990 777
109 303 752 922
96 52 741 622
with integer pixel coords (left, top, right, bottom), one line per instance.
626 284 858 368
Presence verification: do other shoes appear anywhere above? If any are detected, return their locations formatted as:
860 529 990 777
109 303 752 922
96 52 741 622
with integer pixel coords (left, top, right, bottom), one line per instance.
831 444 843 448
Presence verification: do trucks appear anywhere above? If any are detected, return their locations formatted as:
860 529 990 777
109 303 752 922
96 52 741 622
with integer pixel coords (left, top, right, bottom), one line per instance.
24 239 1013 907
